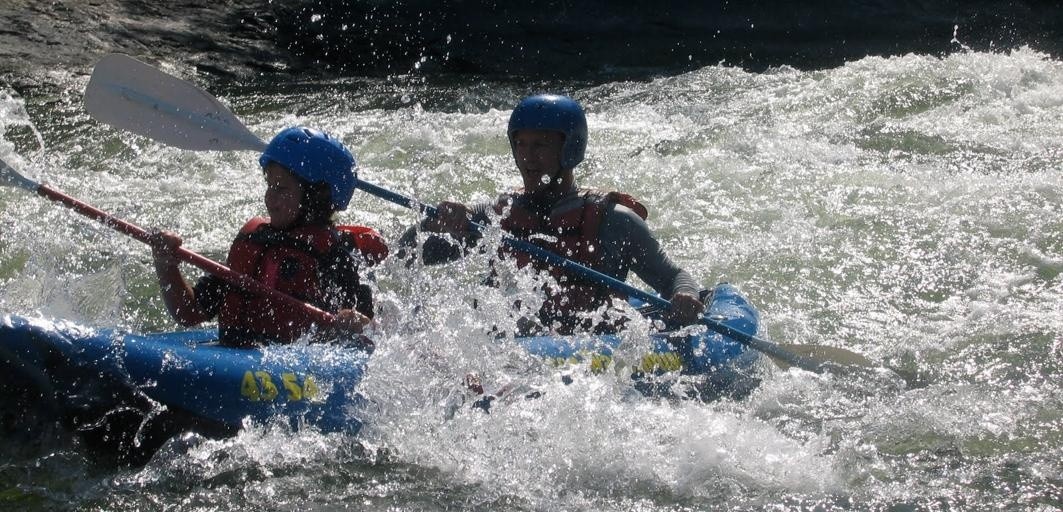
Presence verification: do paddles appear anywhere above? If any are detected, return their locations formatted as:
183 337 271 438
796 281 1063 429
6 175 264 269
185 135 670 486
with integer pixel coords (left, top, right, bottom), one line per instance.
83 54 869 377
0 160 344 329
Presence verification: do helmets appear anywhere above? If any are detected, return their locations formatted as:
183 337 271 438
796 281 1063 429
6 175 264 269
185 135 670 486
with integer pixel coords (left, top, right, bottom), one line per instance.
507 95 586 170
259 128 357 210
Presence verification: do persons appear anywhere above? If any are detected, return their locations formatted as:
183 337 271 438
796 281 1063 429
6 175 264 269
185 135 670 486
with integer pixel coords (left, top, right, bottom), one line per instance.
397 93 706 339
153 125 389 351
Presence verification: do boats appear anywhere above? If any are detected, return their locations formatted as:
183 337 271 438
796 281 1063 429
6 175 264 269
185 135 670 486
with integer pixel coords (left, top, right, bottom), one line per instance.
1 281 758 451
